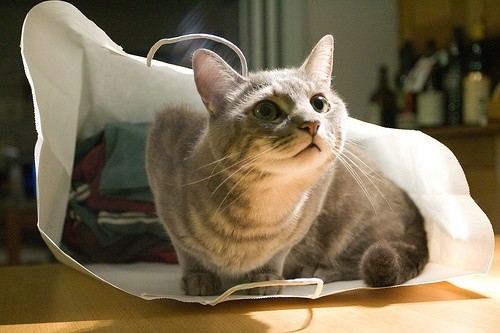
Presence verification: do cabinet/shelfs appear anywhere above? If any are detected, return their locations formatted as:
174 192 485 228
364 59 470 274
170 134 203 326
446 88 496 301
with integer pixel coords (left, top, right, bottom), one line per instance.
397 1 500 235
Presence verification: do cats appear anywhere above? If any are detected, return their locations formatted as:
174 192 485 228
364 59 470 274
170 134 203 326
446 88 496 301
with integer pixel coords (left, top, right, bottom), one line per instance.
144 34 429 297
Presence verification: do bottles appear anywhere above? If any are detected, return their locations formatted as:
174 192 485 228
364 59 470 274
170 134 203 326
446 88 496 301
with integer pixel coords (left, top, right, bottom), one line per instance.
368 67 396 128
393 16 490 129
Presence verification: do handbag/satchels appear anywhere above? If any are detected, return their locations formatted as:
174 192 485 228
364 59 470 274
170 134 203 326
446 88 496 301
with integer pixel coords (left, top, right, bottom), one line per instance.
20 0 496 306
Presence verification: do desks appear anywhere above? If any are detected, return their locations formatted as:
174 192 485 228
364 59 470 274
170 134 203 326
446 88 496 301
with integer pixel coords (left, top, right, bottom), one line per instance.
0 235 500 333
